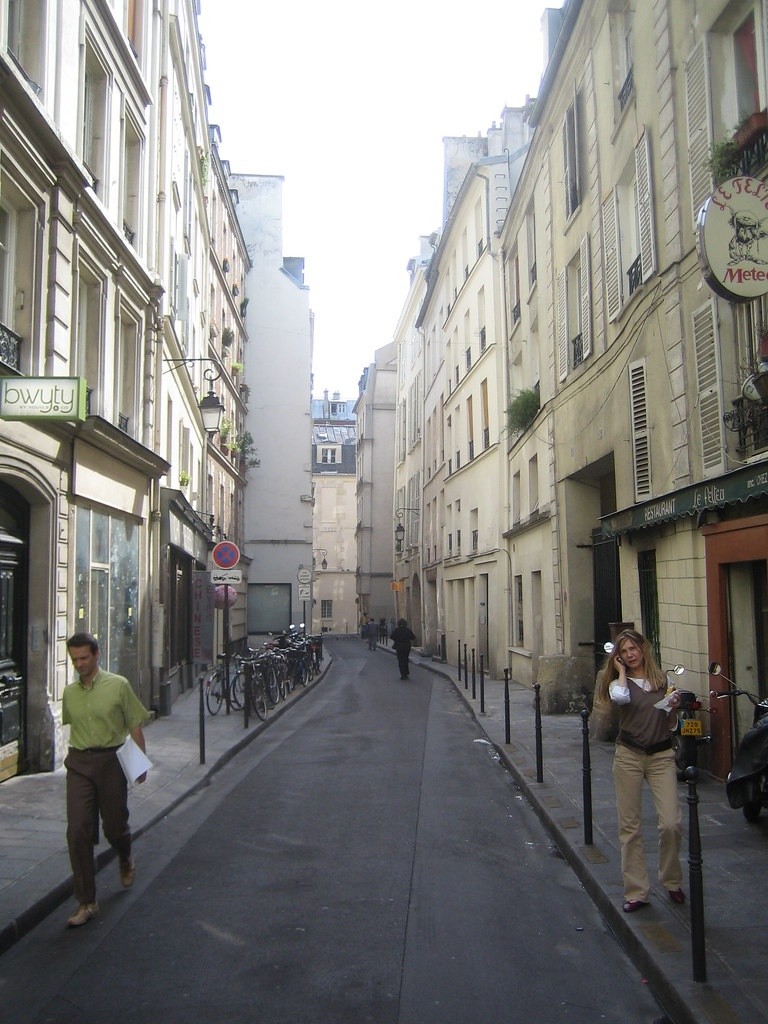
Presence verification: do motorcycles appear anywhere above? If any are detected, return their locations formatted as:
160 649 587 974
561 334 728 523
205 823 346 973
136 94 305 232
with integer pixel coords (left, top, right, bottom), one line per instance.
708 661 768 826
604 642 703 782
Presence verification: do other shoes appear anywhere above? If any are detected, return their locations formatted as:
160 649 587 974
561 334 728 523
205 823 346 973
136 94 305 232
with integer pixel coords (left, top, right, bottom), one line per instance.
669 888 685 905
622 899 644 913
119 855 135 887
400 675 408 680
67 898 101 926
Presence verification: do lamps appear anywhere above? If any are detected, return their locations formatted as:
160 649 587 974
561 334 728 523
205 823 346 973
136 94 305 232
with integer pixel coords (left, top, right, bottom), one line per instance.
162 358 225 433
313 549 328 570
395 507 418 542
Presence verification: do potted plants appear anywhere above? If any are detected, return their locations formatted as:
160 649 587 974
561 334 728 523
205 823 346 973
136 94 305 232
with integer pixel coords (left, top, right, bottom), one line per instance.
219 417 261 476
179 470 191 495
735 320 768 407
221 327 234 357
231 361 244 377
204 195 249 317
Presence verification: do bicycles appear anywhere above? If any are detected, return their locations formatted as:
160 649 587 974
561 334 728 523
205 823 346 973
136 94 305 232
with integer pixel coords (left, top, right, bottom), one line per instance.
205 623 324 721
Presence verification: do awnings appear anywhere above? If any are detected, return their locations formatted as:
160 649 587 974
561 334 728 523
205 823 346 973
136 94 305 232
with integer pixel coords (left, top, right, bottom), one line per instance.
598 460 768 540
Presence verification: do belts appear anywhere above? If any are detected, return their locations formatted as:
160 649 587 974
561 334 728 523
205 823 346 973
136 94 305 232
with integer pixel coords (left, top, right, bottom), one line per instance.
85 745 121 752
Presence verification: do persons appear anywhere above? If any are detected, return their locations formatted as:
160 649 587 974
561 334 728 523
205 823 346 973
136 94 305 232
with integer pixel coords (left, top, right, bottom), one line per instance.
360 613 368 639
366 618 378 651
62 633 150 925
390 618 416 680
598 630 685 912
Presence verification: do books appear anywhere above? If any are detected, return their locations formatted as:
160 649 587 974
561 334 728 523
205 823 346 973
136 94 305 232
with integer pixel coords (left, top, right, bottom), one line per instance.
114 740 155 787
